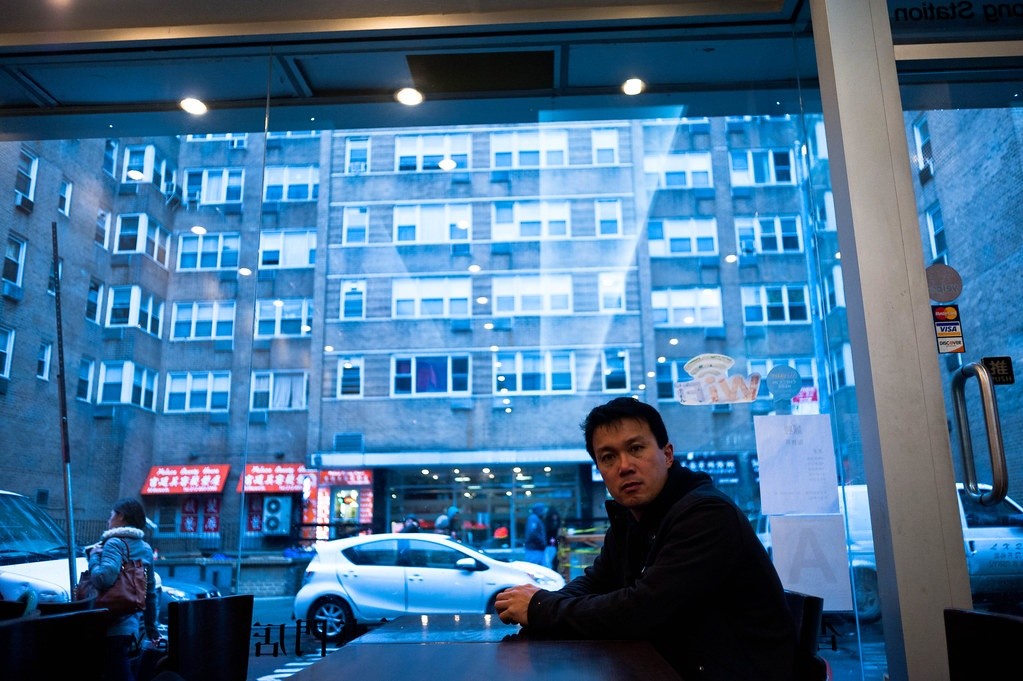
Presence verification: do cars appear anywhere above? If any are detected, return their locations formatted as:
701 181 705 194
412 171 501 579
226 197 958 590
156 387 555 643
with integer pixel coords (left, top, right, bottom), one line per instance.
156 567 223 624
293 530 569 643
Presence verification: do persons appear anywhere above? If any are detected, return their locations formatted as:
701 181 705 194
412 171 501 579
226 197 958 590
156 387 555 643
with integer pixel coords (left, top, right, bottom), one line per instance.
495 396 795 681
434 506 459 533
84 499 163 681
400 512 427 565
523 503 560 569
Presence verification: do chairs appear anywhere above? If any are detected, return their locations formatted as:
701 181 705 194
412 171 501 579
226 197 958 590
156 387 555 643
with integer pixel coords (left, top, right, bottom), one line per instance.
0 606 110 681
944 607 1023 681
164 594 254 681
785 590 824 681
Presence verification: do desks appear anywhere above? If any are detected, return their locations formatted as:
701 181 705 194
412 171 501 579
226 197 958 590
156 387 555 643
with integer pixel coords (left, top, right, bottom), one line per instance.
285 642 619 681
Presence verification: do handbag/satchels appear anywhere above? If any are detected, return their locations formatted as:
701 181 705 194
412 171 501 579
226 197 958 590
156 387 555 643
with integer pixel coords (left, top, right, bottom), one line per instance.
75 536 147 618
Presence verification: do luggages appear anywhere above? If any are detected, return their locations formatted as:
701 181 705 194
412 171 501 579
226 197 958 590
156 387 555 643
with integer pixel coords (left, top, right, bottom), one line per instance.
136 639 169 681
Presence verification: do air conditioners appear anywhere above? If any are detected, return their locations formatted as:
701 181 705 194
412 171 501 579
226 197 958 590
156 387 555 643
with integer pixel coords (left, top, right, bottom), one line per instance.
262 495 292 535
167 182 183 200
449 318 474 332
185 191 200 201
492 316 513 331
448 171 471 183
449 242 473 256
450 396 474 411
490 170 512 182
234 138 247 149
741 239 757 252
492 396 514 409
490 241 513 254
15 194 35 214
350 162 365 171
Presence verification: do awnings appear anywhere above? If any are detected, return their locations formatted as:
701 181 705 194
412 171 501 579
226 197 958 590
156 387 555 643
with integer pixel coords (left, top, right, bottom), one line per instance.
140 464 231 493
237 463 305 492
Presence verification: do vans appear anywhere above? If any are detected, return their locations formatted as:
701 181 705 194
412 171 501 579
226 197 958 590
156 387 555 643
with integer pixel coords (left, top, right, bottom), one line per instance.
0 490 89 605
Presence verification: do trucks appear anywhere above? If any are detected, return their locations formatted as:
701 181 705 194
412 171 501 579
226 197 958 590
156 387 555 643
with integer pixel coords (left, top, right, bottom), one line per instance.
759 482 1023 626
